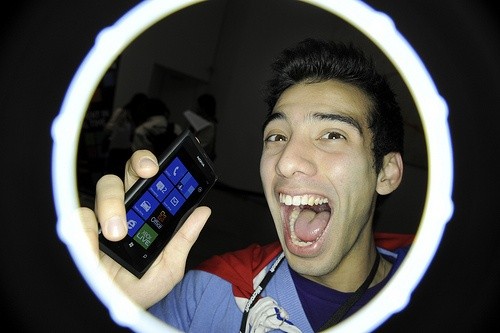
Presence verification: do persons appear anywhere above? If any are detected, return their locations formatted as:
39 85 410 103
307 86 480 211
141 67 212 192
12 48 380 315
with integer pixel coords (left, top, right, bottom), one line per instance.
76 35 418 332
189 92 218 164
94 92 185 203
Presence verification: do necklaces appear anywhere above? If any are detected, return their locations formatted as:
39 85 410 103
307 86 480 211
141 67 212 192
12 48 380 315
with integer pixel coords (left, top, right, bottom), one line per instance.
242 250 381 333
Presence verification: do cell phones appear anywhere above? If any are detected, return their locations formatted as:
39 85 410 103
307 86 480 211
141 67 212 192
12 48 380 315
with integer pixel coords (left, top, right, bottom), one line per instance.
93 129 219 282
